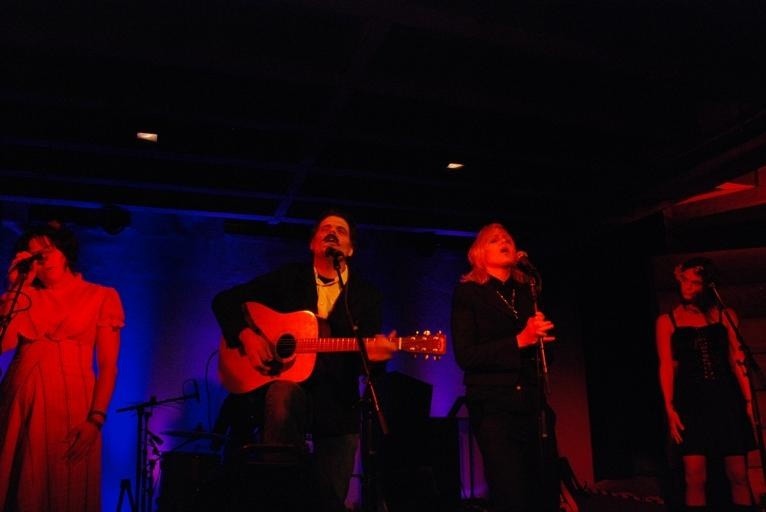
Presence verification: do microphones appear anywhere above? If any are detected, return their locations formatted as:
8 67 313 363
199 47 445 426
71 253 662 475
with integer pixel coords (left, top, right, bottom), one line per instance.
515 248 538 274
695 265 715 287
18 251 48 274
322 245 346 261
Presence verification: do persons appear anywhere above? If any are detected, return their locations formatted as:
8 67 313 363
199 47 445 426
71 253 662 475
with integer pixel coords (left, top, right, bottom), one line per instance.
452 222 559 512
216 208 400 510
0 218 126 509
654 250 760 506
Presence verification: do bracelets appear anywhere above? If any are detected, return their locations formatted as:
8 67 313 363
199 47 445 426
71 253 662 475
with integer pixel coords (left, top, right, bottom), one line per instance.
88 410 108 431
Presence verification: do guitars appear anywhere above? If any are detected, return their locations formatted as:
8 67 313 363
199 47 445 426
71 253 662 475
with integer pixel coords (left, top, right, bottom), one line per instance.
219 302 447 393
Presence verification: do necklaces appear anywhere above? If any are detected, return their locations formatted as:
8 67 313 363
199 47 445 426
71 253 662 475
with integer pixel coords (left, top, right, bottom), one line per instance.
493 284 522 321
